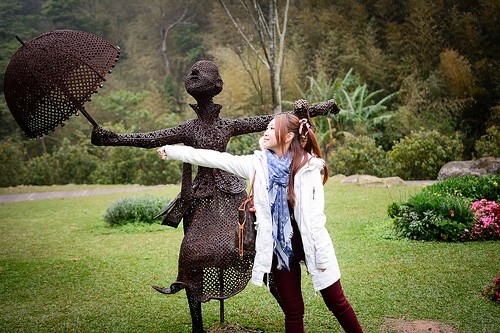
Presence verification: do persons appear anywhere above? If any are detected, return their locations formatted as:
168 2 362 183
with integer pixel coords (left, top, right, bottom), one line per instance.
156 112 364 333
89 59 341 333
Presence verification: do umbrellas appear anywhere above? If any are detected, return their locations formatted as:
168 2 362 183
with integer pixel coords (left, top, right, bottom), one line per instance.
4 28 121 141
